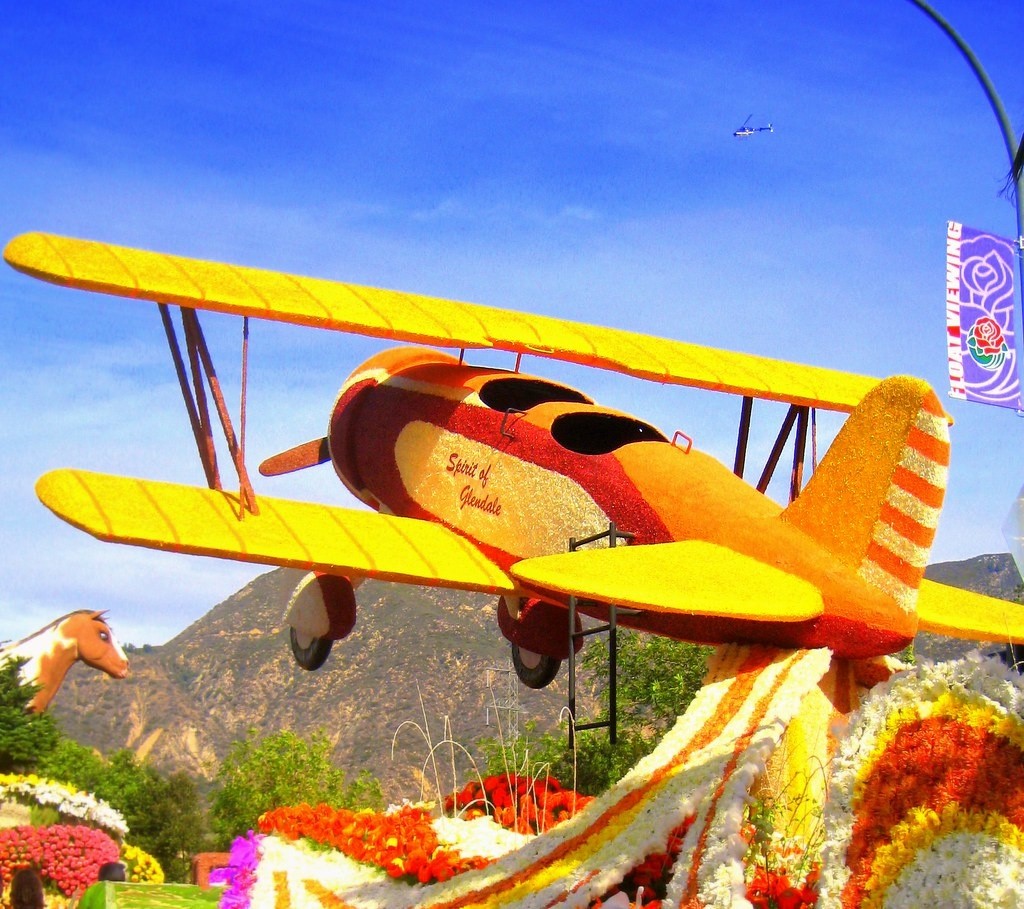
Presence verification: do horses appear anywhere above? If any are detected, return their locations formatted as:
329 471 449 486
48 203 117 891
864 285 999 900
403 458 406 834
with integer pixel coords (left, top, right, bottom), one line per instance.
0 608 130 719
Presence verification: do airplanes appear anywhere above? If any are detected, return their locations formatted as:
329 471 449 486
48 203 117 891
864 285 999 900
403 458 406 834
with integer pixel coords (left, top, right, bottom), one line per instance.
733 115 775 136
0 228 1024 714
